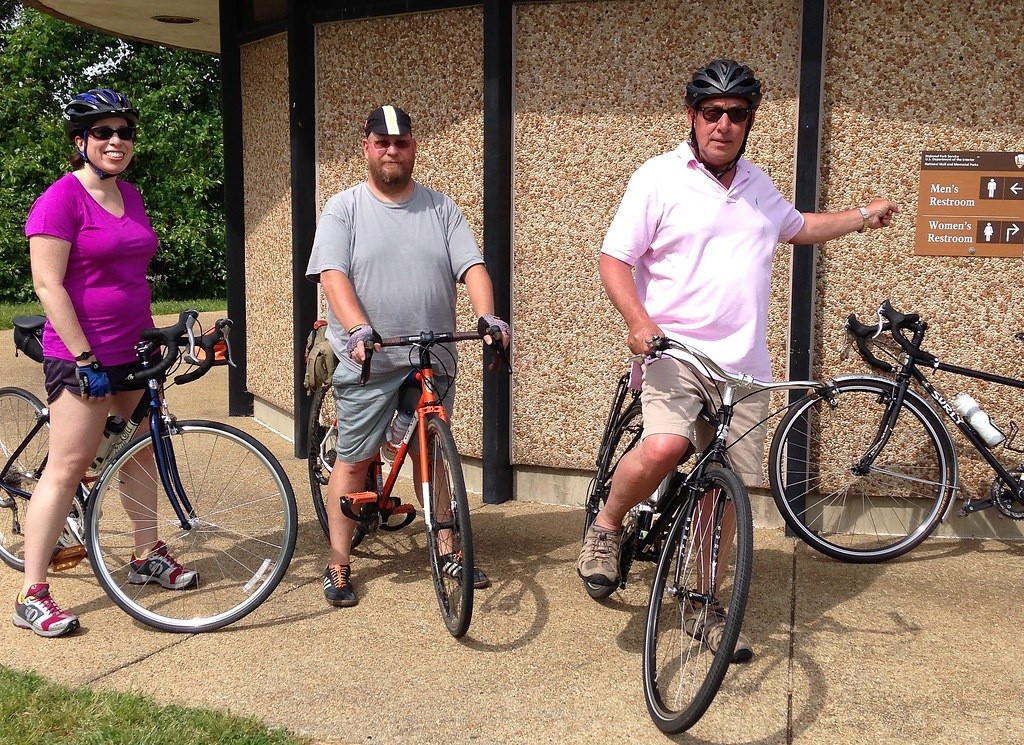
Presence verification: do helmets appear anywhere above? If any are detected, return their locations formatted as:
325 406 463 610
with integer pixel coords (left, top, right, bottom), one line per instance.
685 59 762 114
63 89 139 141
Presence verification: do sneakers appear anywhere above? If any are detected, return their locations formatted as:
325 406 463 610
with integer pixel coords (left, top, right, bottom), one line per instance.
432 549 489 588
682 589 752 663
576 515 623 587
13 582 80 637
126 540 200 590
323 565 357 607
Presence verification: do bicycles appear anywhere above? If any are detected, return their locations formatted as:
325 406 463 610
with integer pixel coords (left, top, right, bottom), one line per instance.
769 298 1024 565
305 317 515 639
0 306 298 635
580 333 842 734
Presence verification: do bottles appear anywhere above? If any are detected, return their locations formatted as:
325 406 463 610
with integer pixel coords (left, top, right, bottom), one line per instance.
954 393 1005 449
381 410 413 466
86 415 126 469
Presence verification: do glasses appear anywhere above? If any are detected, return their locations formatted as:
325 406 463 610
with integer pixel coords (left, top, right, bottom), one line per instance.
86 126 137 141
693 106 752 123
367 137 412 149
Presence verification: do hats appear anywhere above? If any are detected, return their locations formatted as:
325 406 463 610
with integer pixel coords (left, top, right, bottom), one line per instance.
364 106 412 139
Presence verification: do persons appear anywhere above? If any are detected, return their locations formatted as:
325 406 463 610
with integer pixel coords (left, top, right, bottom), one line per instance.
11 87 200 638
573 59 900 664
305 104 511 607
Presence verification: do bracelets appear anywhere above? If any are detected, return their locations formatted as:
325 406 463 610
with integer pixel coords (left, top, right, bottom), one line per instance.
857 207 868 234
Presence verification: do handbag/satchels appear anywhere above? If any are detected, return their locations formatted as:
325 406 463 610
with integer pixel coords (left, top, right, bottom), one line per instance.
303 318 339 397
